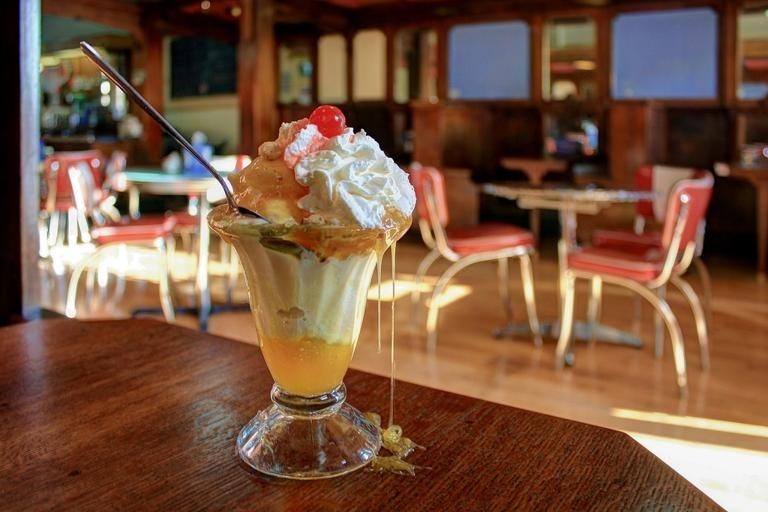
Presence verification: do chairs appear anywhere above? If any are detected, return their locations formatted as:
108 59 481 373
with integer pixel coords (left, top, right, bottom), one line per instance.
554 171 725 386
409 161 546 350
39 151 105 259
65 164 178 322
162 153 251 305
591 165 715 353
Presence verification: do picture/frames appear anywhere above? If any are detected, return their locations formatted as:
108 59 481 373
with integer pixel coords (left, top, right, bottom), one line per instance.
543 17 599 100
735 9 767 102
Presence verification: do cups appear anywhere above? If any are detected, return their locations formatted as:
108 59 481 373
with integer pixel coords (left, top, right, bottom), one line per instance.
207 201 414 482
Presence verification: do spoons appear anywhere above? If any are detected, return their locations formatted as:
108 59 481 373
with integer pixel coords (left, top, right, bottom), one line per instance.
79 40 280 224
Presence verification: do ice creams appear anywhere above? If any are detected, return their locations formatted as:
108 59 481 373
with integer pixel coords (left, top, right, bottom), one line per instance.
206 105 416 396
